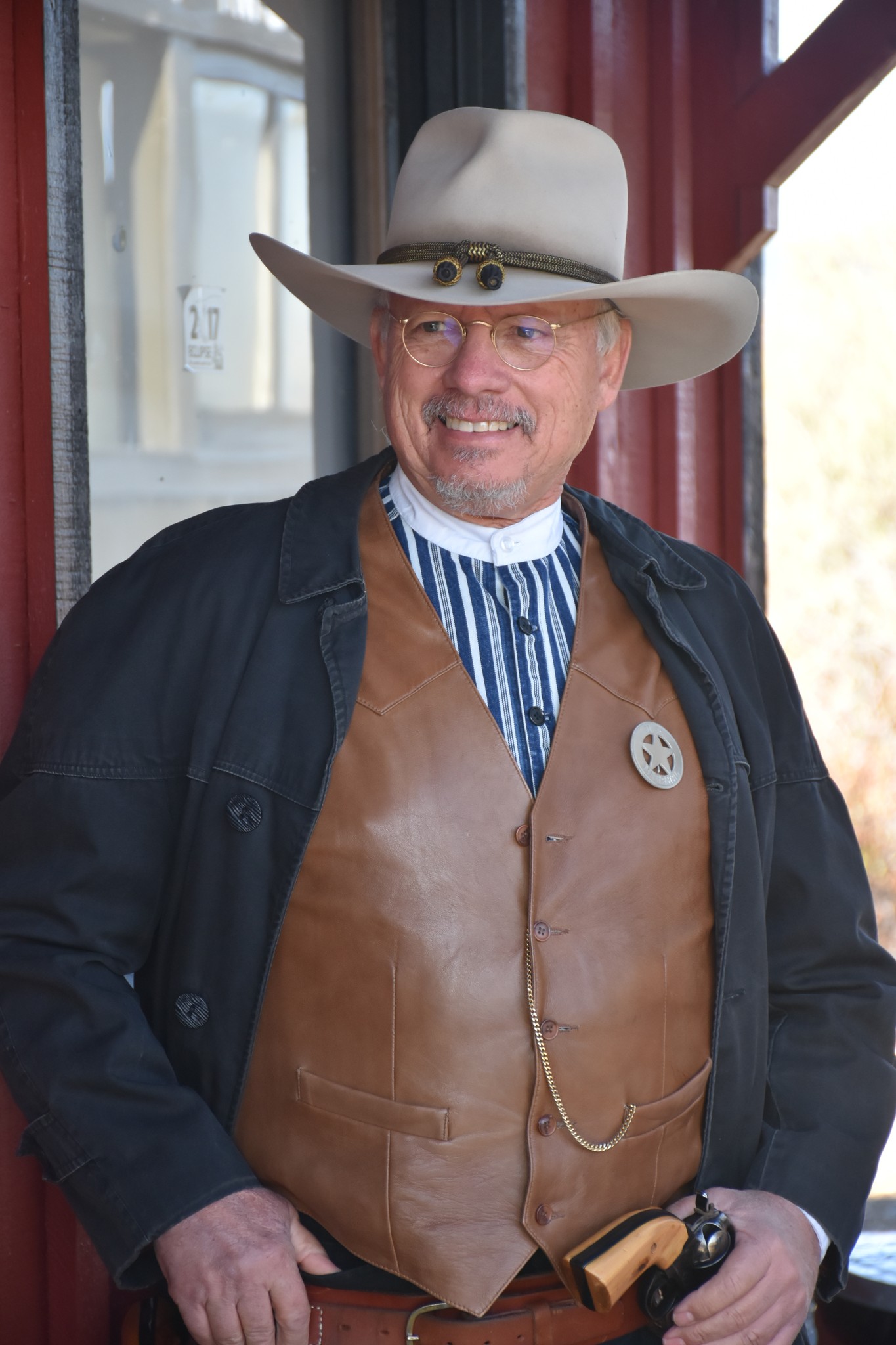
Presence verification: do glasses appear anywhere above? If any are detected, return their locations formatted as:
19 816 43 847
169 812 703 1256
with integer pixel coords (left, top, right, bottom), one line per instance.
375 304 627 373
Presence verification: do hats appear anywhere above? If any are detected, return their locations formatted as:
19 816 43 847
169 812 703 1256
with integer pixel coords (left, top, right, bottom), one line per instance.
249 107 761 393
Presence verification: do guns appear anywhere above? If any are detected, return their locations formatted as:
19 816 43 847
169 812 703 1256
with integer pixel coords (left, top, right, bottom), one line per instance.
561 1190 737 1315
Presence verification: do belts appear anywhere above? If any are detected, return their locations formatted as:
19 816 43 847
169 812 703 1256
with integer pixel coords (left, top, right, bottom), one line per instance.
271 1284 664 1345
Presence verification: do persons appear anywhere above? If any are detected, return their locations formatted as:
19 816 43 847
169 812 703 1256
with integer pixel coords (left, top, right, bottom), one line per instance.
0 125 896 1345
